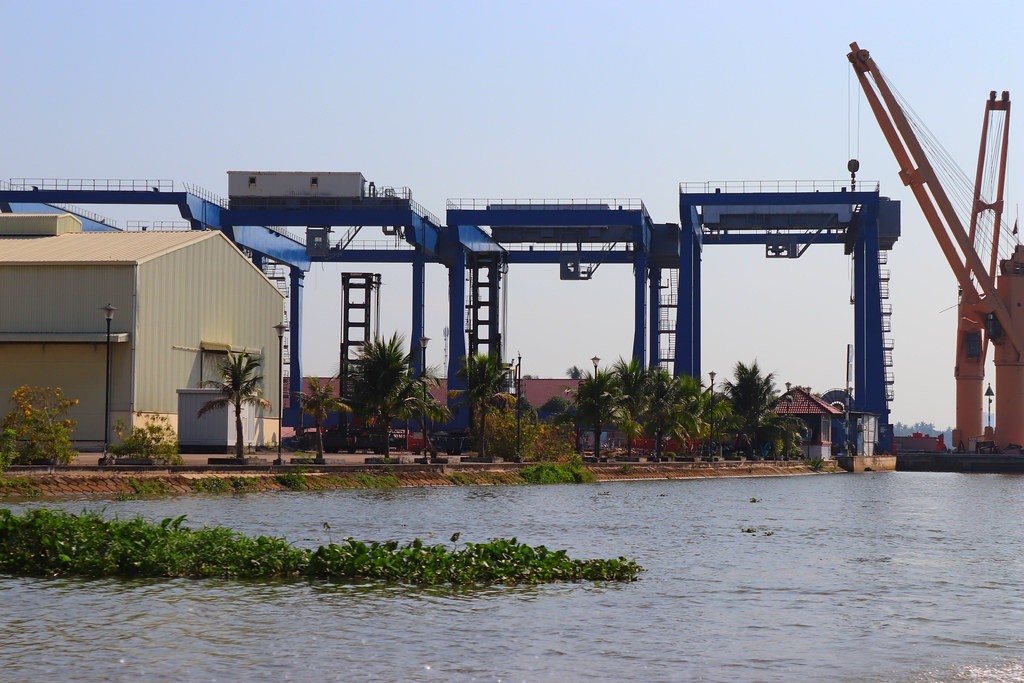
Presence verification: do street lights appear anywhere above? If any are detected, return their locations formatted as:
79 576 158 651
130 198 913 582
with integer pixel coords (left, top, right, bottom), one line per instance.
784 382 792 461
708 370 718 462
804 386 812 460
98 303 118 465
418 335 432 464
273 322 289 465
591 355 601 462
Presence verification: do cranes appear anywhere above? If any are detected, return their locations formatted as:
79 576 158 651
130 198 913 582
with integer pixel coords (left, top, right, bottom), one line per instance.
845 41 1024 454
